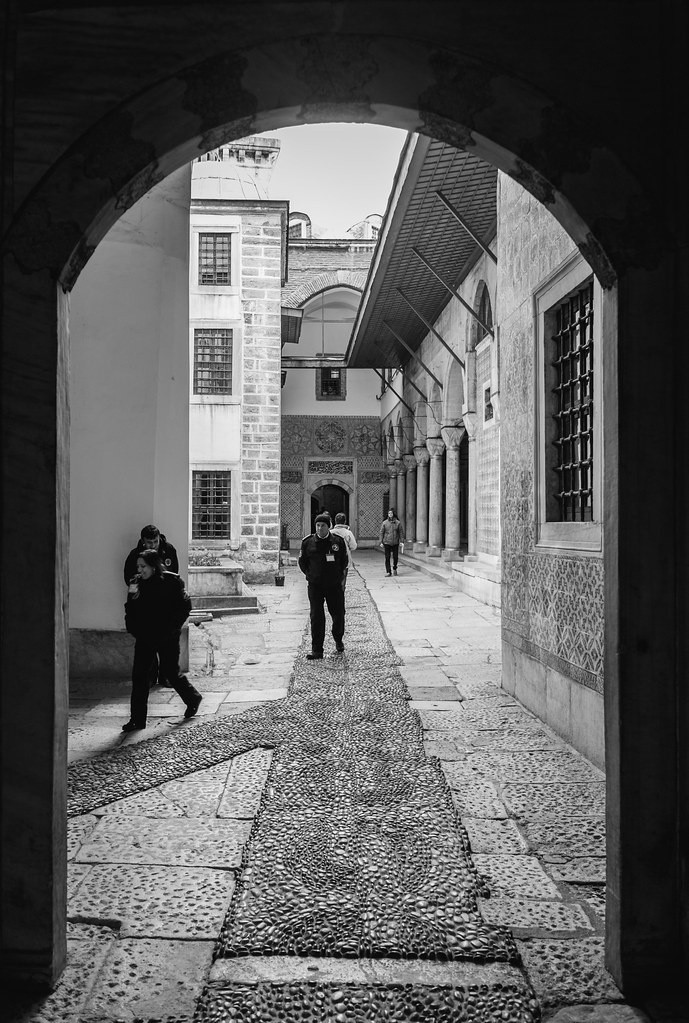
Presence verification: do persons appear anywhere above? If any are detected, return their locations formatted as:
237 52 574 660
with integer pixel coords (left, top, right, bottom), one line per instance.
124 525 182 686
298 513 351 659
327 513 357 595
378 509 405 578
122 549 202 733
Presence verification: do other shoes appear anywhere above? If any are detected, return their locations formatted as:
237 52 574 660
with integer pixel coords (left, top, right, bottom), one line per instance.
393 571 397 576
184 694 202 718
122 719 146 732
336 638 344 652
306 652 323 659
385 572 391 577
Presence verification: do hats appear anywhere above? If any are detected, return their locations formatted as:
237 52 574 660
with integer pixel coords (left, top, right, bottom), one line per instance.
314 514 330 528
